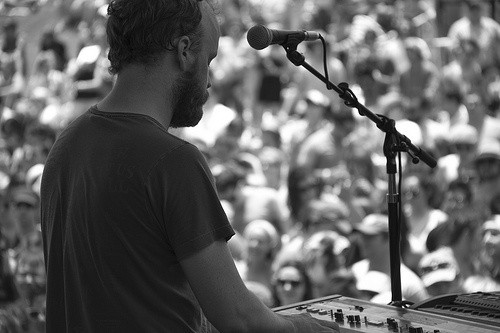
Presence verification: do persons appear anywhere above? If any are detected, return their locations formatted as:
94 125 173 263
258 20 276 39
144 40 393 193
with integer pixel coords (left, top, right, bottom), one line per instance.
0 0 500 333
40 0 340 333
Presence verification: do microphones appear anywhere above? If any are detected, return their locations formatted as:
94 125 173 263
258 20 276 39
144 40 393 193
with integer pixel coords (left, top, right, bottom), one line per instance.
247 24 321 50
